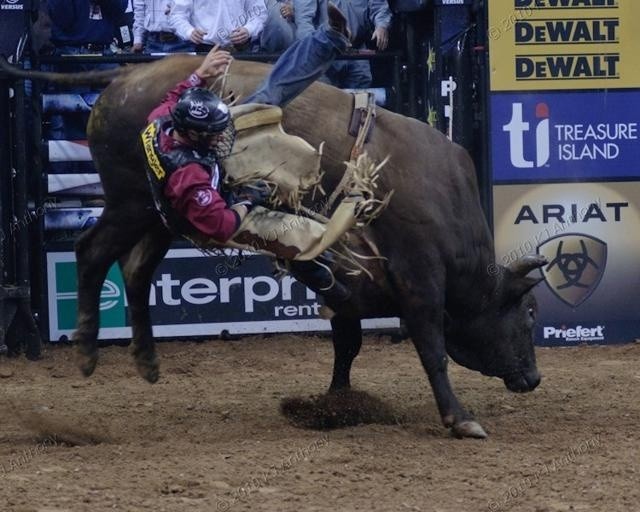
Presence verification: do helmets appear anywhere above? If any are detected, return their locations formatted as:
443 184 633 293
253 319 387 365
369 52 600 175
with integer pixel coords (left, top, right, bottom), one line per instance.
174 87 235 163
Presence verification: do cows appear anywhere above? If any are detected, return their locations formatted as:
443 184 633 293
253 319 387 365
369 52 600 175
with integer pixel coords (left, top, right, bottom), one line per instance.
0 55 549 439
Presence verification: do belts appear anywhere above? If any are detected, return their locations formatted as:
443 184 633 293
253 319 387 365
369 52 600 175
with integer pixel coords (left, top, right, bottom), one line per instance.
60 41 103 51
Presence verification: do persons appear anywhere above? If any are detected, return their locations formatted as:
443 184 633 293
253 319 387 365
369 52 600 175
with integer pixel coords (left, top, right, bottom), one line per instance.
138 1 390 304
1 0 473 148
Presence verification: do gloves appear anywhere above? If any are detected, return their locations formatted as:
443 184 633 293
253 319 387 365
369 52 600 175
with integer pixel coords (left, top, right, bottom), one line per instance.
234 182 268 213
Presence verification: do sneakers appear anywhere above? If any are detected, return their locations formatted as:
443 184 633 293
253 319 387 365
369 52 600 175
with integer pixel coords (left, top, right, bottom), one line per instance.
327 0 353 39
326 280 353 304
